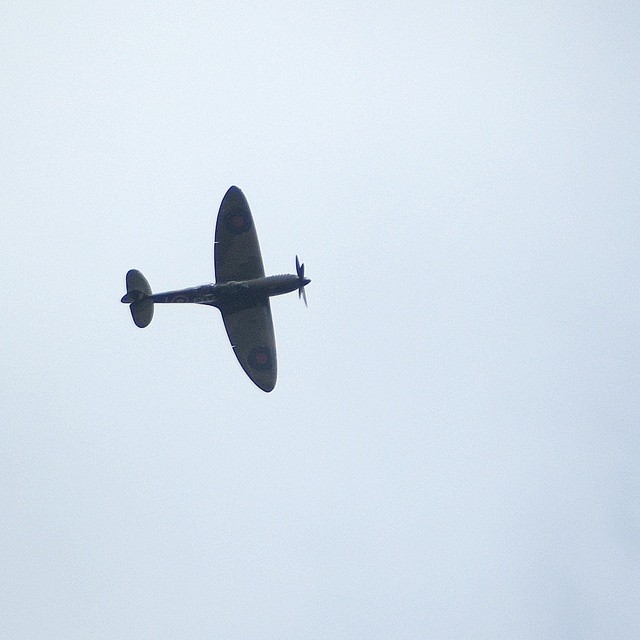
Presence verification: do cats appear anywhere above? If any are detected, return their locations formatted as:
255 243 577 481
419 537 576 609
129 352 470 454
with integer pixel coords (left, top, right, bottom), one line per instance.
121 186 311 392
